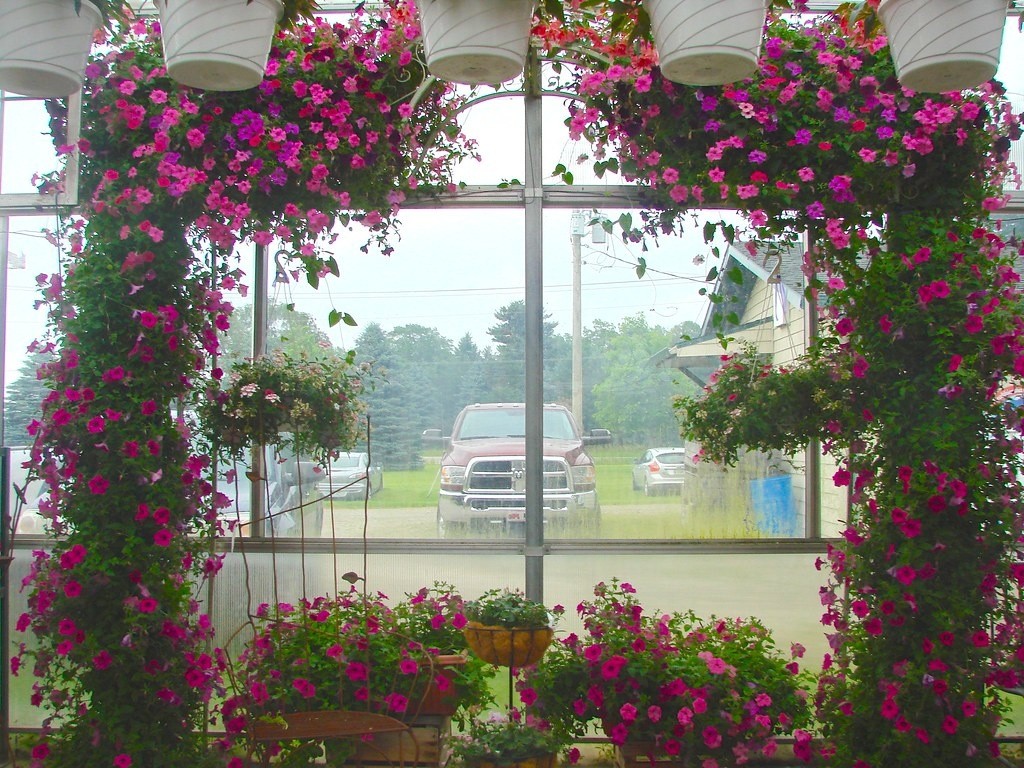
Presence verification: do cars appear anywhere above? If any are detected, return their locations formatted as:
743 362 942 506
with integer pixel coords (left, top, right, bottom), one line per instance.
171 409 323 538
632 447 685 497
7 446 75 535
316 452 383 501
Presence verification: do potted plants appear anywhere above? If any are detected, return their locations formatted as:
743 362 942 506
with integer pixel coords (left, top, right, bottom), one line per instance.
462 587 553 667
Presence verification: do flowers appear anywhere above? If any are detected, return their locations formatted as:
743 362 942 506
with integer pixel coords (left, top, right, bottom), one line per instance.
383 0 565 65
807 0 1024 78
73 0 135 45
377 585 466 655
613 0 808 51
276 0 324 39
439 706 581 763
202 356 387 477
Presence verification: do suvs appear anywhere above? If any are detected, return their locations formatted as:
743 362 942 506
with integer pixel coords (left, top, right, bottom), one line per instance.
421 403 613 541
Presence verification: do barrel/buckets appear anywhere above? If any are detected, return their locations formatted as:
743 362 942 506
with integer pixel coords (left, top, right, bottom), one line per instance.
750 476 798 536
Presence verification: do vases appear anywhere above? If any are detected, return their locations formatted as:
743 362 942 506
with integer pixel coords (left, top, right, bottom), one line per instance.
641 0 773 86
419 0 539 85
152 0 284 91
385 651 469 718
1 0 103 97
468 750 557 768
322 716 443 768
274 411 317 435
877 0 1010 92
